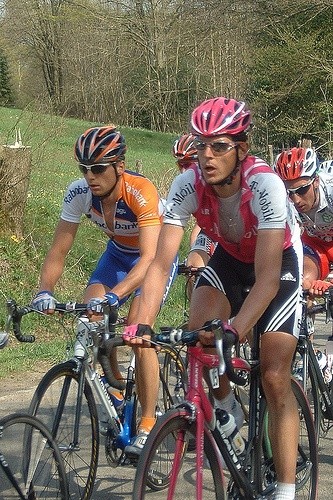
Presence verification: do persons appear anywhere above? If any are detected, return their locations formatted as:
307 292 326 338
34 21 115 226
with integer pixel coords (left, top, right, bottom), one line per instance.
272 147 333 383
172 134 219 297
122 97 302 500
33 125 178 457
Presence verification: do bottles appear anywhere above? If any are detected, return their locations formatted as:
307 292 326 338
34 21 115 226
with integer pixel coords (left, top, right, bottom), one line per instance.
215 408 245 456
315 349 332 384
96 362 125 410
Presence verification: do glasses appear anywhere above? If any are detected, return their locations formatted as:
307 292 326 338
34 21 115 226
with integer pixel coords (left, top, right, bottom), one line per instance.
287 177 316 199
176 161 196 170
80 161 120 174
194 138 246 155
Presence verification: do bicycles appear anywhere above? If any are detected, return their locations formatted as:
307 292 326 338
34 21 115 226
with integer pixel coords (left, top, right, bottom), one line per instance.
102 317 320 500
0 415 71 500
178 263 333 480
12 299 187 500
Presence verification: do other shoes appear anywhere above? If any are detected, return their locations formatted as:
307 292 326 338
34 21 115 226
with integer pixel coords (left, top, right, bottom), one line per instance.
294 350 327 380
100 395 126 435
206 408 245 471
124 428 148 462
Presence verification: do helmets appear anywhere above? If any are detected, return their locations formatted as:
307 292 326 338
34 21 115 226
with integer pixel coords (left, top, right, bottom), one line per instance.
75 127 126 164
191 97 252 139
273 147 320 179
172 134 199 161
319 160 333 178
